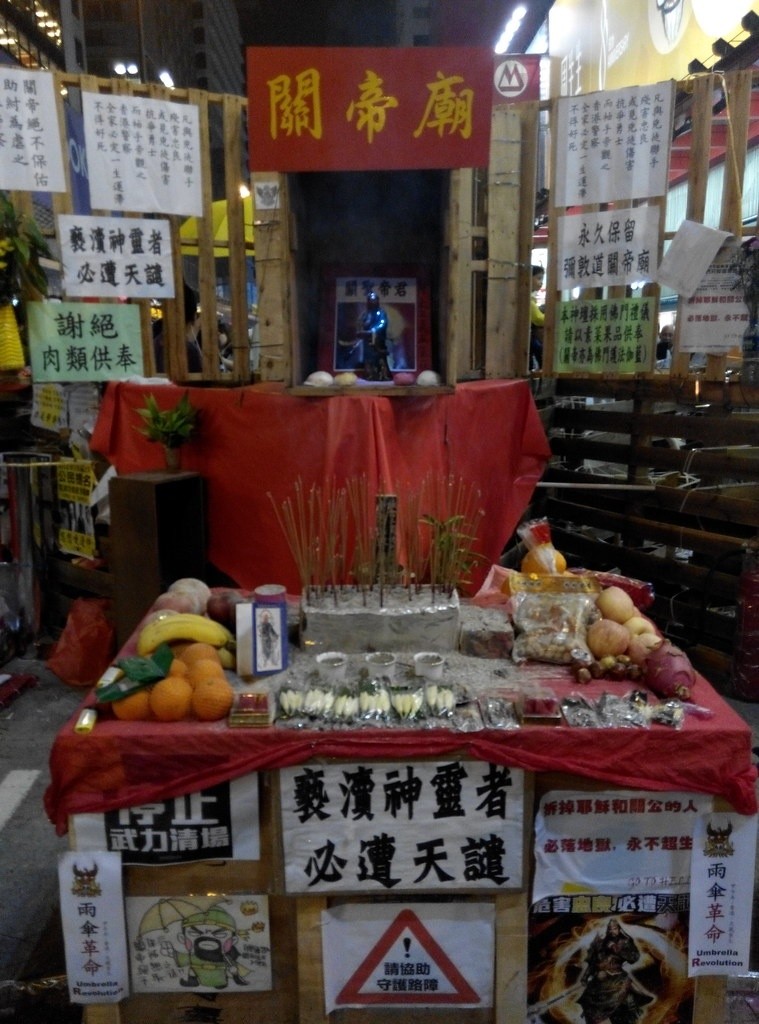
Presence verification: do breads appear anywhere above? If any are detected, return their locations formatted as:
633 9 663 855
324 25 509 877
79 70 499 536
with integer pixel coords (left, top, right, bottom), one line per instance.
306 370 439 387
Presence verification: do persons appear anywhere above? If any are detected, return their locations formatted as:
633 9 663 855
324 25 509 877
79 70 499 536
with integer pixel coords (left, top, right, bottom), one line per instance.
529 265 547 373
152 278 260 373
656 325 675 360
355 291 392 381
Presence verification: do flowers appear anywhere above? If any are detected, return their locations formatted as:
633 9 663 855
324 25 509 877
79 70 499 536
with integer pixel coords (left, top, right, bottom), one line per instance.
0 190 59 306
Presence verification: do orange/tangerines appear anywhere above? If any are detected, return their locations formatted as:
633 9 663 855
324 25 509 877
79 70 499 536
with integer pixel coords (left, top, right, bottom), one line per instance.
112 642 233 721
502 546 573 594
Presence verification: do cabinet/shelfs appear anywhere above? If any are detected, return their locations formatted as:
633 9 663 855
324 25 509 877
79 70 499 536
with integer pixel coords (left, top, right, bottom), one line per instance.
42 566 759 1023
107 471 211 654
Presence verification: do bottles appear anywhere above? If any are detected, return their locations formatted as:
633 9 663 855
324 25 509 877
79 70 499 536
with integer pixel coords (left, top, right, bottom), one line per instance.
252 586 289 675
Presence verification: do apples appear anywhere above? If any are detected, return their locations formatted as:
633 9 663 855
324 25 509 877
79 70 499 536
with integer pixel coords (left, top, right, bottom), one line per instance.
151 578 245 628
587 586 662 664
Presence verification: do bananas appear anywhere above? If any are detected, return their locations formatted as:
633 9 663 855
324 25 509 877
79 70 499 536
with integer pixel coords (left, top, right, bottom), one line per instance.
136 613 236 670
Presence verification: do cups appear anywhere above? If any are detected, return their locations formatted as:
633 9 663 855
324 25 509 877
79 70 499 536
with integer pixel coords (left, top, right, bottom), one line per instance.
413 651 445 680
316 650 350 682
366 653 398 683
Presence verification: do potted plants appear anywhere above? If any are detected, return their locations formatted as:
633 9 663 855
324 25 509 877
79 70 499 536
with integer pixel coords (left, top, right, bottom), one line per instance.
131 389 200 473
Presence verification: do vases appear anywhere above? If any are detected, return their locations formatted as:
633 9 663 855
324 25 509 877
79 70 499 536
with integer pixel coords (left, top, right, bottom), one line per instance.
0 306 25 371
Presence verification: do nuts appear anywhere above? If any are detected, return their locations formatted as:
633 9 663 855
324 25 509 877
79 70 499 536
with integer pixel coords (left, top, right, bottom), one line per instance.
517 603 584 664
